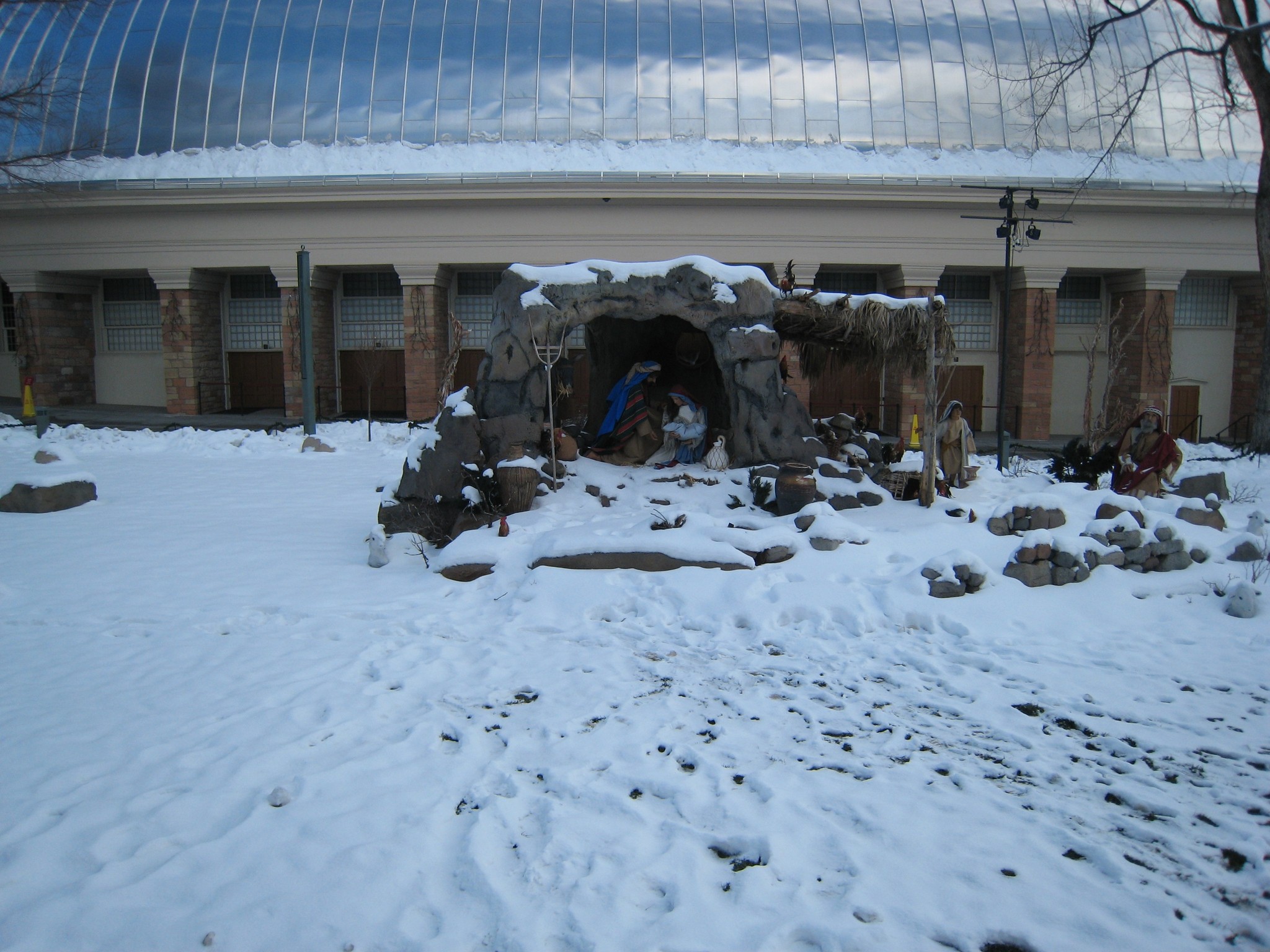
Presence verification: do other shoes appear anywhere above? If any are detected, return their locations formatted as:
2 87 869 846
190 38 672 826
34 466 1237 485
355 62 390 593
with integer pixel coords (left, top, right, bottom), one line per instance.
654 463 665 470
666 460 679 467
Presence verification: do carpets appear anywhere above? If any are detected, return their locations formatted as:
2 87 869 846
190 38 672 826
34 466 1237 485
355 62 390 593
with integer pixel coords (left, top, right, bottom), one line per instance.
210 407 265 415
873 430 894 437
335 411 405 418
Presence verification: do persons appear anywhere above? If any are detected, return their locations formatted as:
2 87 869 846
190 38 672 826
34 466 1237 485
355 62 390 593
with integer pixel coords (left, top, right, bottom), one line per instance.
661 384 708 466
1112 406 1182 499
937 401 976 490
579 358 666 466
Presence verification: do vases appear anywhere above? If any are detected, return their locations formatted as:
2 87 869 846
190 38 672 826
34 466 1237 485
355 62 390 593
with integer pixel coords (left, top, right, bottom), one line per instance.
500 443 540 514
775 462 817 515
706 435 729 469
